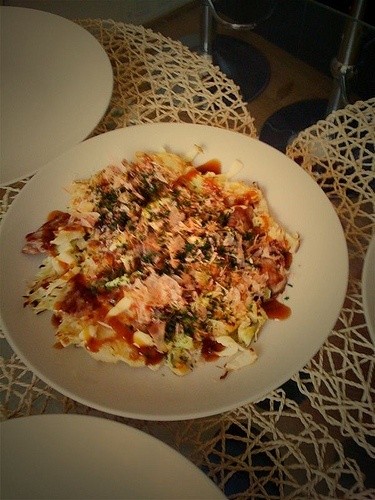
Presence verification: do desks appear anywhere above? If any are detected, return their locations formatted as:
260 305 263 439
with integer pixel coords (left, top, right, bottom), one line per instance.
0 16 375 500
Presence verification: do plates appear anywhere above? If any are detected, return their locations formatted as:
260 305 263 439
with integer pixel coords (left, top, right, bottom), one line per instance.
0 123 349 422
361 233 375 348
0 5 113 188
1 414 231 500
